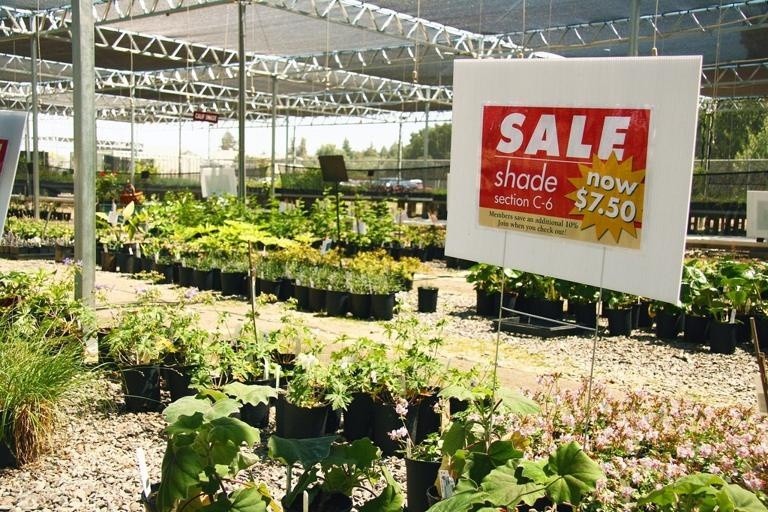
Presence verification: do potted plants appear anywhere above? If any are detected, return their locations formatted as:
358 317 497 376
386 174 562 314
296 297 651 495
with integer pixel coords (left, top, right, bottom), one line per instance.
0 184 768 352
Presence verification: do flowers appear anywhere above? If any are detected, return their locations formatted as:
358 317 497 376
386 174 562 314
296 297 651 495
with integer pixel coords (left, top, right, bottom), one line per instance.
0 263 767 511
95 168 118 201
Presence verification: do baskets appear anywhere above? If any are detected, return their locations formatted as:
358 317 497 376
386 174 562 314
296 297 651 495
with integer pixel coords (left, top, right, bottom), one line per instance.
120 184 143 204
